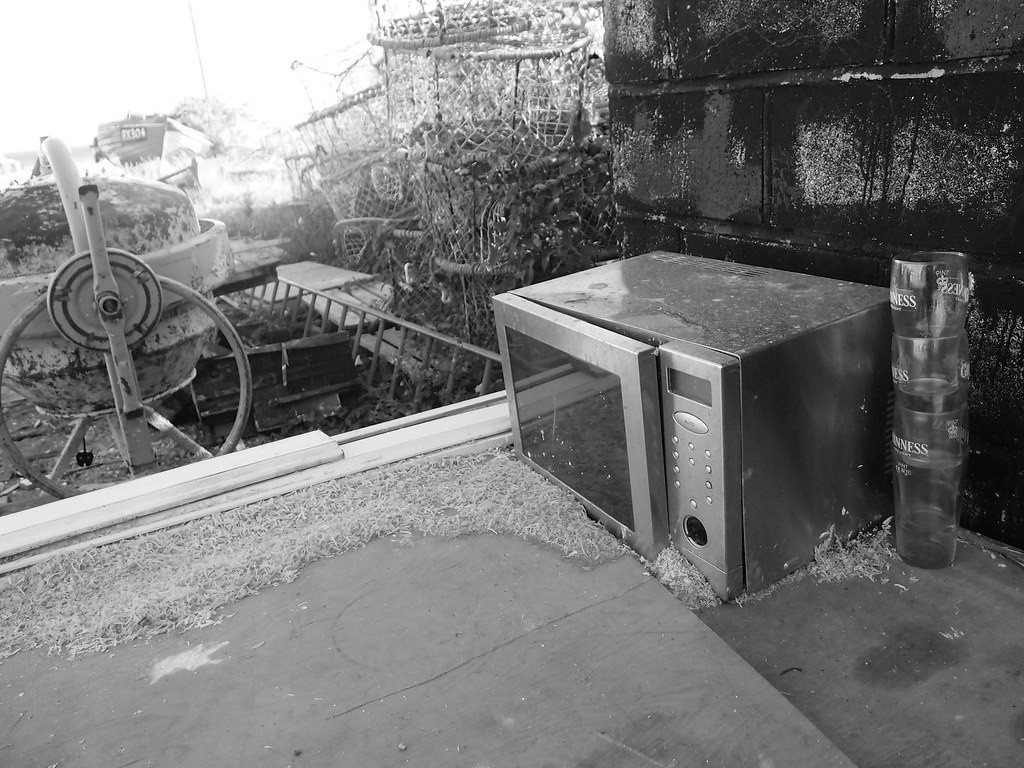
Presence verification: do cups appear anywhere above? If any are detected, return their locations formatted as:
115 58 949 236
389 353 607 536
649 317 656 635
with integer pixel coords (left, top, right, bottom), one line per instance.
889 251 970 568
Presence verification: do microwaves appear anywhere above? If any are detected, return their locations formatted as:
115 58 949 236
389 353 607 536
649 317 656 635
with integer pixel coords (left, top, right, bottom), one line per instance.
492 247 887 607
